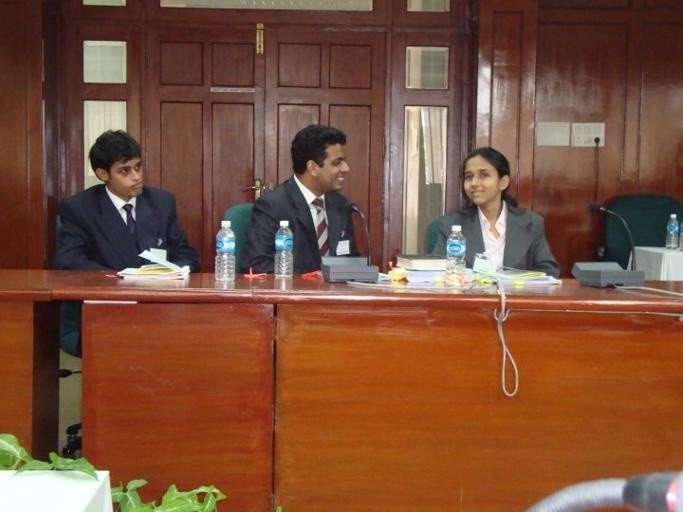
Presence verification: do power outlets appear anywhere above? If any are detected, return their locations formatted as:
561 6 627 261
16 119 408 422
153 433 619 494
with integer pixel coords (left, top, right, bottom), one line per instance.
572 121 604 149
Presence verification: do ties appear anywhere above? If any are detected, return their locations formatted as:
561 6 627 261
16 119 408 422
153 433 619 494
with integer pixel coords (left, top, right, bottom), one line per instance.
310 198 329 258
121 203 135 239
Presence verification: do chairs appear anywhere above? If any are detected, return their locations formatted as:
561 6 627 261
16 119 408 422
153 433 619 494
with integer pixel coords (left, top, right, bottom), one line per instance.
596 194 682 270
220 204 254 274
53 214 86 458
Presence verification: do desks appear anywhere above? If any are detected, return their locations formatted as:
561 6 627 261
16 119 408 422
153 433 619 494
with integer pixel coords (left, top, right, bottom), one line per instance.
1 267 683 511
630 247 683 282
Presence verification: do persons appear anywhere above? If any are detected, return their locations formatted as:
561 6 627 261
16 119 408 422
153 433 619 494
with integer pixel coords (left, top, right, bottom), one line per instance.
239 123 360 274
431 147 560 279
51 128 200 359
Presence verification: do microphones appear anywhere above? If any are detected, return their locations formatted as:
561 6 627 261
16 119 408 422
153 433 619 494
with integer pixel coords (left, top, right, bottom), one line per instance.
589 203 636 270
345 201 371 266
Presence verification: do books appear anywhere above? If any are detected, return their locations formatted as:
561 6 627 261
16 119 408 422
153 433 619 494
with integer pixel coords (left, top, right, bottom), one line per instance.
395 253 448 270
116 249 190 280
474 265 544 279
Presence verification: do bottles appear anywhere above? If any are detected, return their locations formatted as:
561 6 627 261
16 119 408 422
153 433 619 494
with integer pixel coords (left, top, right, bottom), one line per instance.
665 213 683 251
445 225 466 288
215 220 236 282
274 220 294 280
